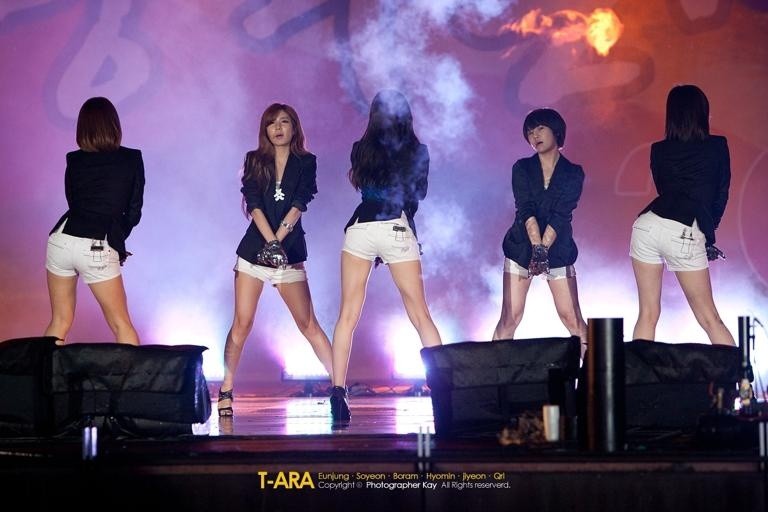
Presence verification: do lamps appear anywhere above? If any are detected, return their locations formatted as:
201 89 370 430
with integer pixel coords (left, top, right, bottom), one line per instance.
278 357 336 400
386 351 444 399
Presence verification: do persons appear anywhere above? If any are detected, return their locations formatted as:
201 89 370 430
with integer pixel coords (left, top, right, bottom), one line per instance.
628 82 739 347
41 97 148 348
328 88 446 415
493 105 588 357
218 99 333 420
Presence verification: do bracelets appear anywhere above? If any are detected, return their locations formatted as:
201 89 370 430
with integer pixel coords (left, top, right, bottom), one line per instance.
280 220 294 232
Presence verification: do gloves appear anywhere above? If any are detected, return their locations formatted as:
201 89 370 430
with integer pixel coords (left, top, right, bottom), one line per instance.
255 239 291 271
524 242 553 278
701 244 728 265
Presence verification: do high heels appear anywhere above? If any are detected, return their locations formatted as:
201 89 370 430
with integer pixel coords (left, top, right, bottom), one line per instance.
214 385 237 419
328 383 357 424
